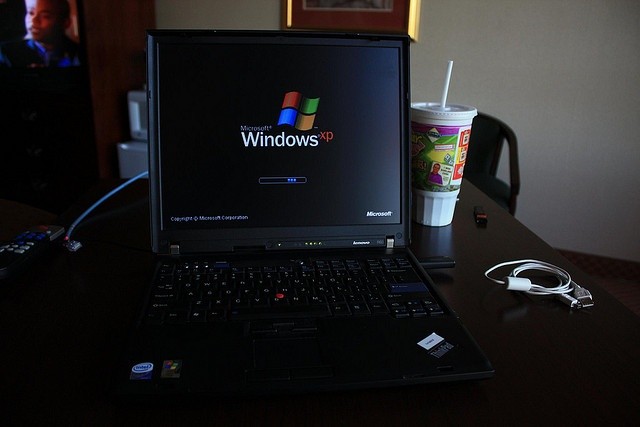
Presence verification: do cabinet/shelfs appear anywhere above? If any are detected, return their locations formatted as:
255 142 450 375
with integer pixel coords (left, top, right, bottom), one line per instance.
0 88 91 195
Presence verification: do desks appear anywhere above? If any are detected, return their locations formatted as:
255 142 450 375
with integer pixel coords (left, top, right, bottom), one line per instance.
1 176 639 425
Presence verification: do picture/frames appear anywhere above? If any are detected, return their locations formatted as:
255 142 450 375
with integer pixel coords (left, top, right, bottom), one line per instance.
279 0 420 42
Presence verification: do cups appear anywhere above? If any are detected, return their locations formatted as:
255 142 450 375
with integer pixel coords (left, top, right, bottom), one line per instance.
409 101 478 228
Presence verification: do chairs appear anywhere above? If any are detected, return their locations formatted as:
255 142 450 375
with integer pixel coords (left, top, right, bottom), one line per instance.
462 111 521 215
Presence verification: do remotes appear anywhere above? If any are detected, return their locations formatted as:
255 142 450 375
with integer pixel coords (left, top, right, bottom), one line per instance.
0 223 65 279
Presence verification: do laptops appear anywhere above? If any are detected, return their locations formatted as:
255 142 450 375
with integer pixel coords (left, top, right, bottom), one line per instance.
107 29 497 402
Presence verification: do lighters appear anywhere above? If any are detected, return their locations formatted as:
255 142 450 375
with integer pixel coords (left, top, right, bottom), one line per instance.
473 205 488 224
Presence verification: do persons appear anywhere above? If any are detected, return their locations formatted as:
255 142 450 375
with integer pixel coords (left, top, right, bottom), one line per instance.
429 164 442 184
8 0 82 72
